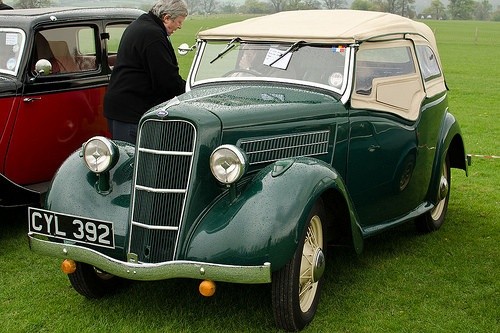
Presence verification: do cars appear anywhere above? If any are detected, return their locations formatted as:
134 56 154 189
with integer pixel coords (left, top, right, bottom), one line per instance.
29 10 473 333
0 7 151 223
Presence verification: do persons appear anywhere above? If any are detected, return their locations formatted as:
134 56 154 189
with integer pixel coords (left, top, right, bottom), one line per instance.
28 32 61 76
102 0 186 146
227 45 266 78
